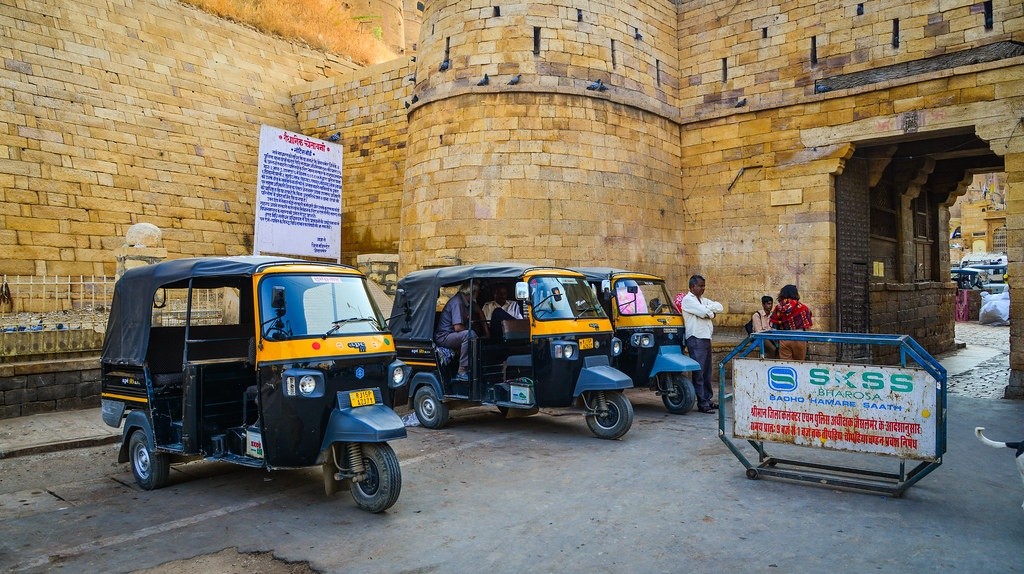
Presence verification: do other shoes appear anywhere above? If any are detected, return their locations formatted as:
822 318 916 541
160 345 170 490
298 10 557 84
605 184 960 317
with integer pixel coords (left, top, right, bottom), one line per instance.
456 372 472 382
698 406 715 414
709 402 719 409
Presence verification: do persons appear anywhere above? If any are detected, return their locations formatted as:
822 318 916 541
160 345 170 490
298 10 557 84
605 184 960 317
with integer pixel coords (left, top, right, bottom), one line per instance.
768 284 813 360
482 286 524 338
434 279 490 381
681 275 723 414
752 296 777 359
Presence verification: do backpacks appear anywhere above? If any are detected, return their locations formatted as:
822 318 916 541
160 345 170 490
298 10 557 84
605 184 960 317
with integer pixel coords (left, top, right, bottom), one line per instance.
745 311 761 342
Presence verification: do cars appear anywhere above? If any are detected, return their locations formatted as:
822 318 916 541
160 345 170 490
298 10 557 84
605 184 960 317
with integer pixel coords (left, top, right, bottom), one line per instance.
950 252 1010 295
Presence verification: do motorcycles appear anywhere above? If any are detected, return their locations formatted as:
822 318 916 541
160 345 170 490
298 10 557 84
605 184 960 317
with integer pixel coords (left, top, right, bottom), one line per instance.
385 262 635 440
567 266 702 415
100 255 413 514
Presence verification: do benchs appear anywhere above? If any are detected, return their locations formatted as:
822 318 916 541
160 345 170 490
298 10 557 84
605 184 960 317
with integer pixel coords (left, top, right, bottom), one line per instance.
144 325 248 396
502 319 531 367
431 308 487 363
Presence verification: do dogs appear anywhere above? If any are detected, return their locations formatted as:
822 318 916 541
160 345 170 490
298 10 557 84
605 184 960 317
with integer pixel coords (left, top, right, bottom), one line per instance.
973 426 1024 508
